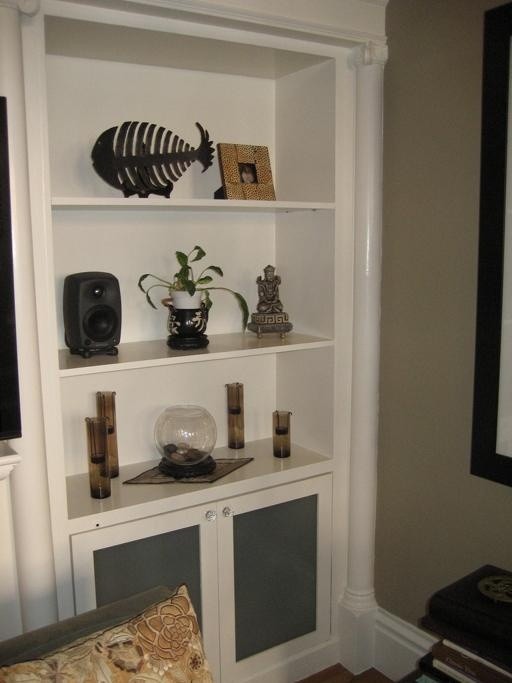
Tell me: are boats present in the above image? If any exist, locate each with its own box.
[63,270,123,359]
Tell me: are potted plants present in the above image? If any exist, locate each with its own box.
[217,142,278,201]
[472,3,511,490]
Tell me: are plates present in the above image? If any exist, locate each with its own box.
[18,0,388,682]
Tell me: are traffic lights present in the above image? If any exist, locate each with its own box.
[2,583,221,682]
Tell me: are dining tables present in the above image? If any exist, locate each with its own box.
[141,247,249,351]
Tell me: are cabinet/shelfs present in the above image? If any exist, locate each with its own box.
[95,389,119,478]
[85,416,111,499]
[271,409,292,457]
[226,382,245,448]
